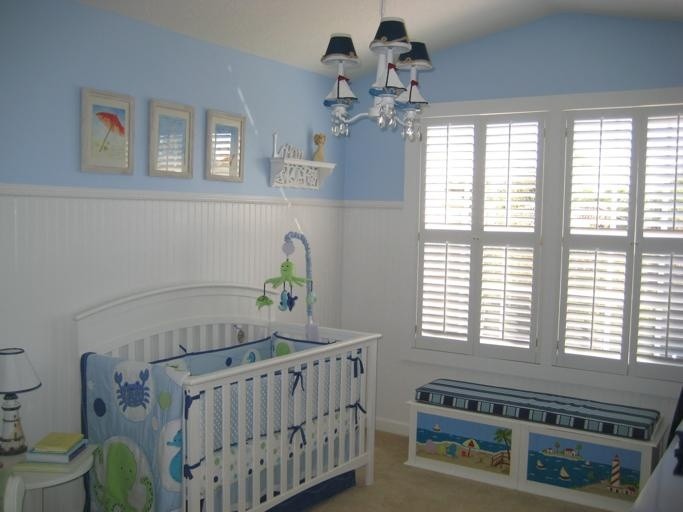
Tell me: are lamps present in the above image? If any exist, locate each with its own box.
[0,346,43,456]
[317,0,434,145]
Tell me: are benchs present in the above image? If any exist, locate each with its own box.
[402,376,672,512]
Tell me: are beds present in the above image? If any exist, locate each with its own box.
[79,313,380,512]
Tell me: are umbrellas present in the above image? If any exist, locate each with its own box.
[463,439,479,457]
[95,109,126,153]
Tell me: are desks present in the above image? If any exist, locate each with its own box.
[0,449,93,511]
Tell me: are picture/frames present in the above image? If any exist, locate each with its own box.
[77,85,247,184]
